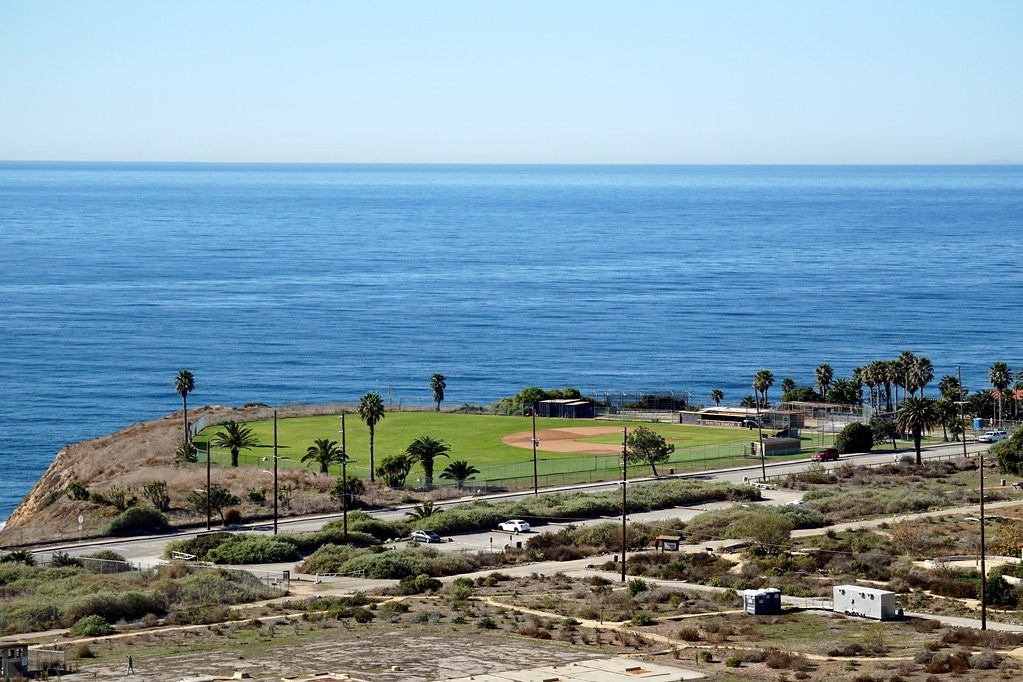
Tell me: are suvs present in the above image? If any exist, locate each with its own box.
[811,447,839,462]
[979,430,1008,443]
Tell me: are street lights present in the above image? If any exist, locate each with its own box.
[744,421,768,481]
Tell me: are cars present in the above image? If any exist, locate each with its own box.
[411,529,441,544]
[498,520,532,532]
